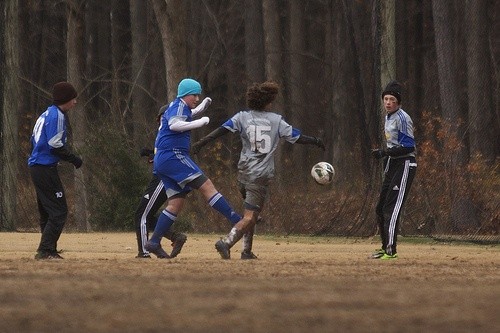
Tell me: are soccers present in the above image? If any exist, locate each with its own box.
[310,161,335,186]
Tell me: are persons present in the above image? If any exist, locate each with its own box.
[369,80,419,264]
[131,102,187,259]
[142,78,261,257]
[29,81,82,261]
[191,80,324,261]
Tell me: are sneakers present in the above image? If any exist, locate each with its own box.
[370,247,397,260]
[170,233,187,257]
[37,251,64,259]
[241,251,257,259]
[145,240,170,258]
[216,240,230,260]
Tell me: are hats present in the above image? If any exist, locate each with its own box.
[382,82,402,104]
[177,79,201,98]
[52,81,77,106]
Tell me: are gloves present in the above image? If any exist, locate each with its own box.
[371,148,388,160]
[73,156,83,169]
[315,136,327,151]
